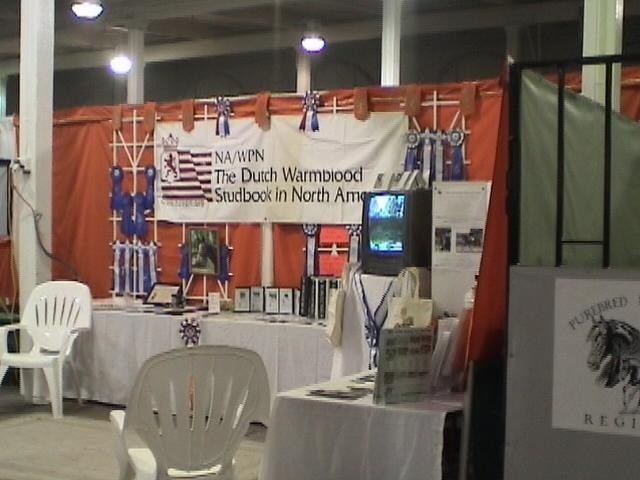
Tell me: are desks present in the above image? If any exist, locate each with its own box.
[22,305,331,423]
[257,371,462,480]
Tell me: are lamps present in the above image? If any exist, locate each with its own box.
[110,51,132,74]
[71,0,102,20]
[299,18,326,52]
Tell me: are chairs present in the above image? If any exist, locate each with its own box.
[1,281,96,421]
[108,345,270,480]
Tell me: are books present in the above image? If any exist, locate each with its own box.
[300,276,343,319]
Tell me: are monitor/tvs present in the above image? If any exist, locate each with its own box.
[361,190,432,277]
